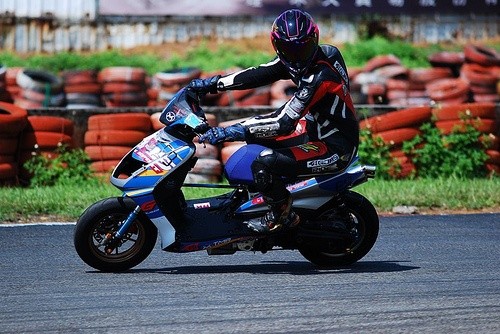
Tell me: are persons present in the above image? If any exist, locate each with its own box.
[188,9,360,233]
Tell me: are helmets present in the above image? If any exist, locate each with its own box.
[270,11,319,77]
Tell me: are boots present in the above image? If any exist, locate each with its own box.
[248,196,299,233]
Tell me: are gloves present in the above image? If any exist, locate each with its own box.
[188,74,222,98]
[198,121,245,146]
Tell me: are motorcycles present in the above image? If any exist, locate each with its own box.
[72,81,380,272]
